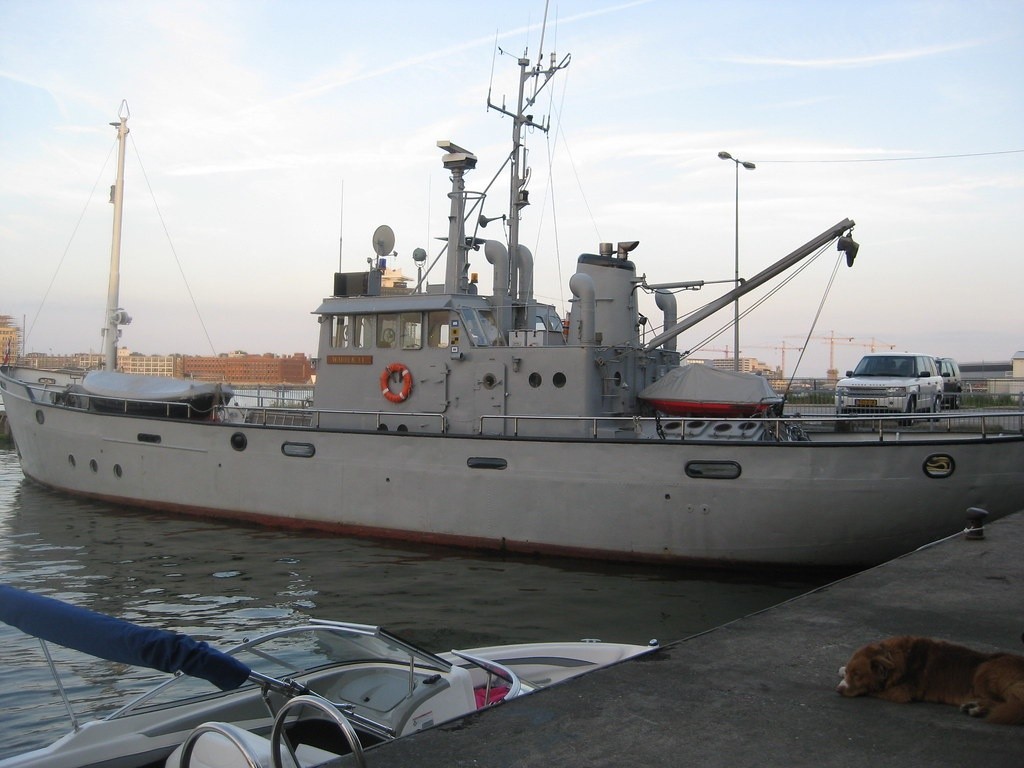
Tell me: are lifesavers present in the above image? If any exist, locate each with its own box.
[380,362,413,403]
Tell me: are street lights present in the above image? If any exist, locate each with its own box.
[716,151,756,374]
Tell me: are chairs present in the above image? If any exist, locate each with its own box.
[164,722,341,768]
[898,361,909,371]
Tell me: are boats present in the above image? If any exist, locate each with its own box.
[1,580,665,768]
[81,369,235,421]
[0,1,1024,571]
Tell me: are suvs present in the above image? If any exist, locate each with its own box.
[834,353,945,426]
[934,357,963,409]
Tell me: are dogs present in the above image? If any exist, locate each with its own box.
[834,635,1024,725]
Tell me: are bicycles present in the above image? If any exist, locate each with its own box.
[760,402,811,442]
